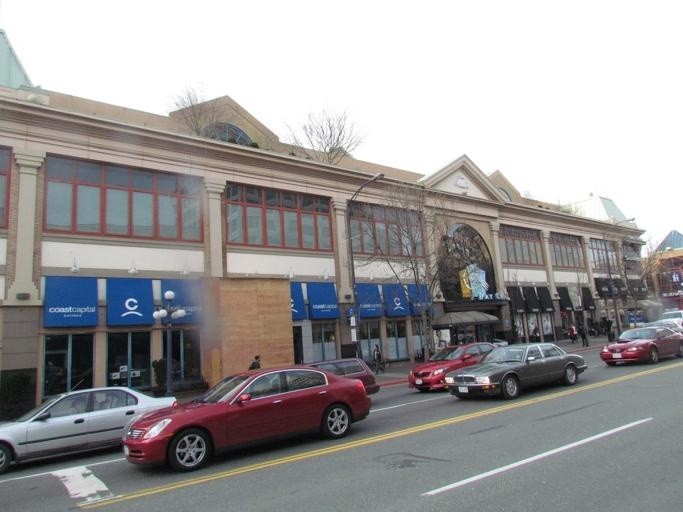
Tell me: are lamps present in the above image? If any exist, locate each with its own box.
[69,254,139,277]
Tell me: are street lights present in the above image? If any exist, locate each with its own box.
[151,287,186,398]
[344,170,387,359]
[601,216,637,337]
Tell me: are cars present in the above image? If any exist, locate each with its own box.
[121,363,372,472]
[598,310,682,366]
[0,386,178,475]
[45,350,205,396]
[406,341,496,393]
[441,343,587,402]
[297,358,380,395]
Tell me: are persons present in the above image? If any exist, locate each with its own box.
[248,355,260,370]
[250,377,272,399]
[74,396,87,413]
[567,323,579,343]
[371,344,383,375]
[577,323,589,347]
[433,330,449,352]
